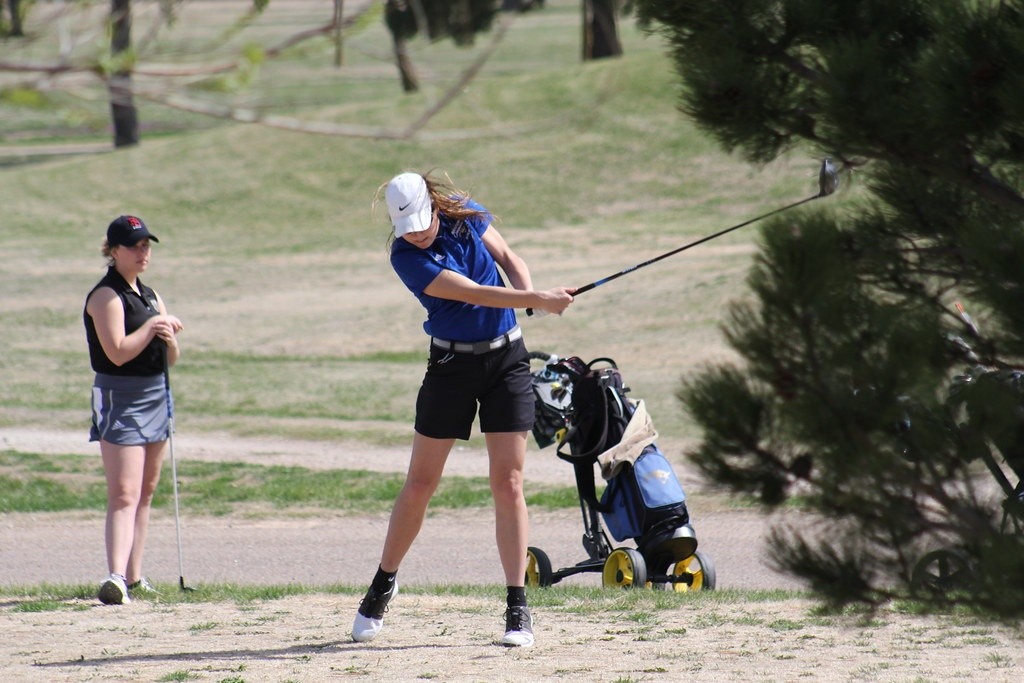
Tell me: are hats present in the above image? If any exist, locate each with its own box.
[107,215,159,248]
[384,172,433,238]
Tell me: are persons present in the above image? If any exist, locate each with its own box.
[84,215,186,604]
[352,172,580,648]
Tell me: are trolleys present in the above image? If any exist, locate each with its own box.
[524,350,716,592]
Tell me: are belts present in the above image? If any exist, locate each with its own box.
[428,324,523,356]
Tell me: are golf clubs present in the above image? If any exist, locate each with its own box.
[160,331,197,593]
[525,160,837,319]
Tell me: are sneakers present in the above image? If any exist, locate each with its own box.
[500,606,534,648]
[124,580,163,601]
[97,578,131,605]
[350,578,399,642]
